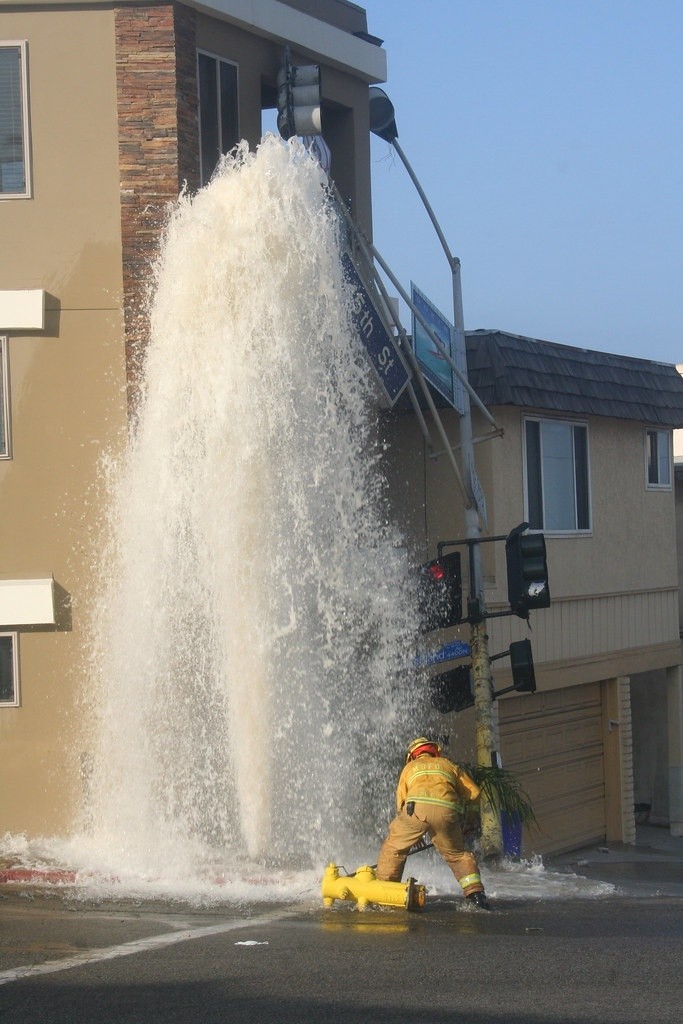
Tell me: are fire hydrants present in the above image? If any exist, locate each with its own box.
[319,863,425,910]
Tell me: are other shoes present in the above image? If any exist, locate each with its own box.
[473,892,496,909]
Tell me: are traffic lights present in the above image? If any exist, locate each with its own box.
[507,528,550,615]
[510,640,537,692]
[431,667,470,712]
[420,552,465,629]
[275,59,322,140]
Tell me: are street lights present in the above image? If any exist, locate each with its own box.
[369,84,502,862]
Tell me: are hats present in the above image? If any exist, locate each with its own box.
[406,737,442,763]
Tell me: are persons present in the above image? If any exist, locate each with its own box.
[375,736,496,911]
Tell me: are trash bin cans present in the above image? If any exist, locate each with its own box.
[459,805,522,863]
